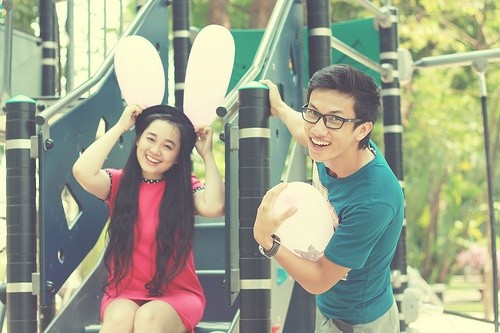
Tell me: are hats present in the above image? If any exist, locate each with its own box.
[135,104,198,152]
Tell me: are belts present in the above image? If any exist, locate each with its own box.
[332,319,353,333]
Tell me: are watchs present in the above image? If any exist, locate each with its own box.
[258,234,281,260]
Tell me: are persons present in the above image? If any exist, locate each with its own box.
[252,62,407,333]
[71,101,226,333]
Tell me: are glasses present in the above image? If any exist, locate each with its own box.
[301,104,365,129]
